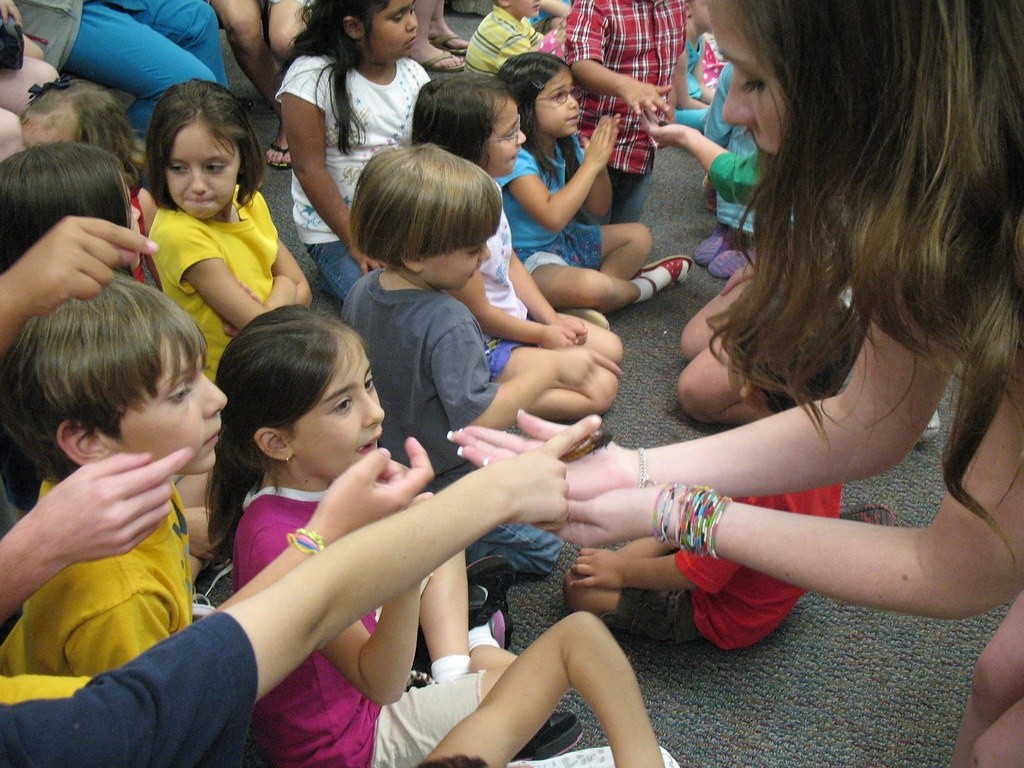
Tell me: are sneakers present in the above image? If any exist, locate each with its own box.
[511,712,584,761]
[466,554,515,651]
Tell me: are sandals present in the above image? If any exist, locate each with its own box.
[631,256,693,295]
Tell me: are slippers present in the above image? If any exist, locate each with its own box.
[429,33,468,55]
[422,49,466,72]
[268,143,292,170]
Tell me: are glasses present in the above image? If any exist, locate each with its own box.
[534,85,582,104]
[482,114,520,141]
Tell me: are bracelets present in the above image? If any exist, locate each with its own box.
[652,481,733,561]
[287,528,326,555]
[638,446,649,488]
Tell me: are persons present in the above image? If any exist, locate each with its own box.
[1,137,231,628]
[422,610,667,767]
[441,0,1024,766]
[206,307,579,768]
[3,412,600,768]
[637,0,942,439]
[1,0,685,418]
[0,272,434,680]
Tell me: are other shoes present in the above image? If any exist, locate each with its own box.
[694,222,737,266]
[835,503,895,527]
[708,245,756,278]
